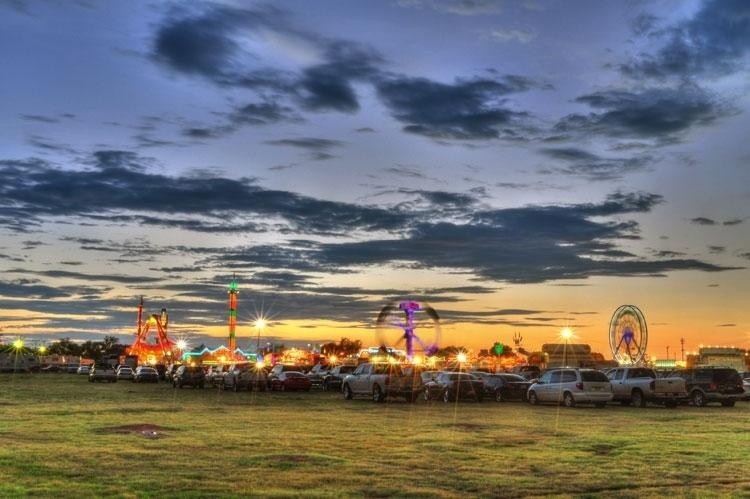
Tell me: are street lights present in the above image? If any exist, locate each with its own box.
[560,325,575,367]
[254,318,268,352]
